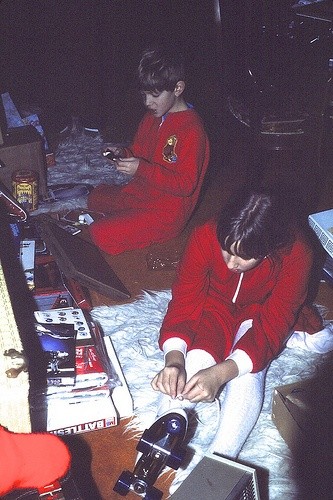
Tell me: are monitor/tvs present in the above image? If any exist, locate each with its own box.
[39,221,130,301]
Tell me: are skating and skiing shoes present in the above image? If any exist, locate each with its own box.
[112,409,188,500]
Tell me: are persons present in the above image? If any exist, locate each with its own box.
[112,189,325,500]
[88,46,210,256]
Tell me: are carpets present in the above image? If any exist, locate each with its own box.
[27,132,133,217]
[90,288,333,500]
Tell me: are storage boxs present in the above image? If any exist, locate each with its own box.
[0,125,48,195]
[272,376,333,459]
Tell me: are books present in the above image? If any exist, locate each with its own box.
[30,322,123,437]
[308,209,333,283]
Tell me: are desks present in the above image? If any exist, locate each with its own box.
[308,208,333,305]
